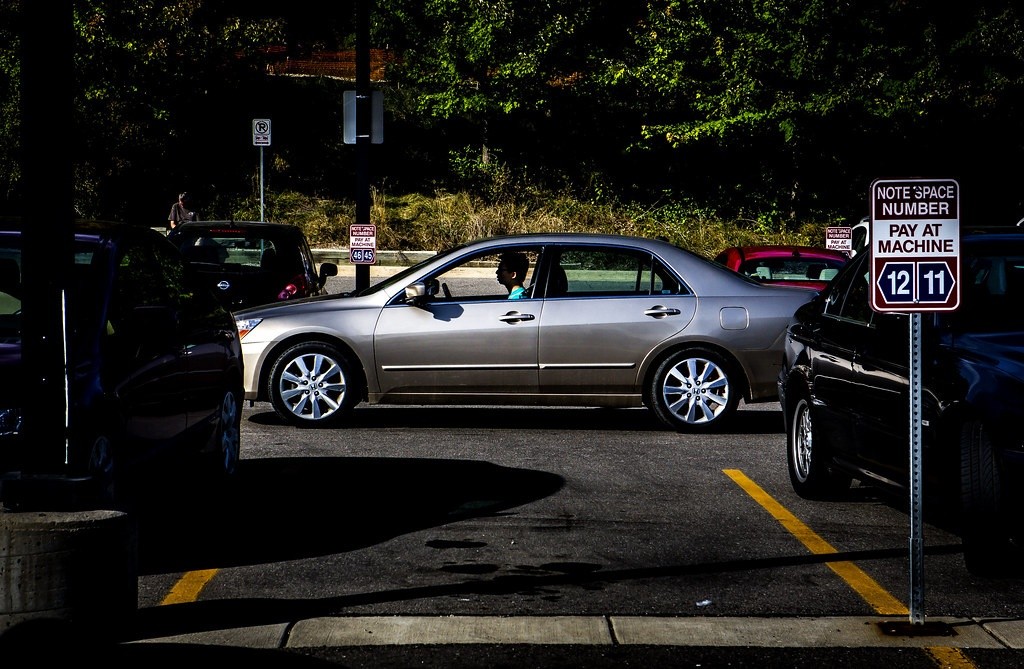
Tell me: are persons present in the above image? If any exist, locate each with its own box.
[167,192,199,228]
[495,253,533,301]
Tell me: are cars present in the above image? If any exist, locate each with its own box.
[709,245,869,293]
[163,220,338,323]
[0,219,246,507]
[847,218,871,263]
[231,226,822,431]
[774,241,1024,569]
[957,212,1023,263]
